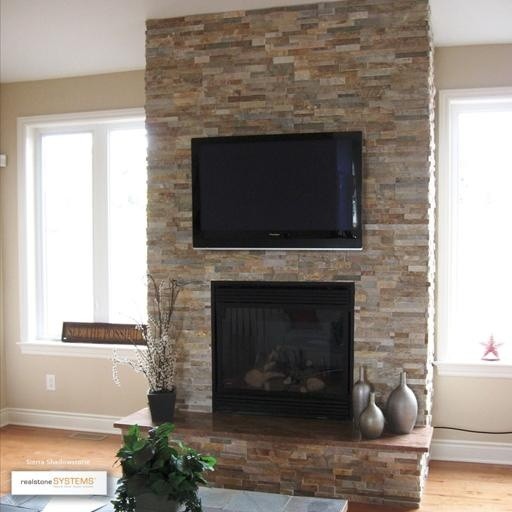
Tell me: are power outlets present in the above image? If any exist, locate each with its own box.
[46,374,55,391]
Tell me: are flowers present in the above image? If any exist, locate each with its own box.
[109,273,207,395]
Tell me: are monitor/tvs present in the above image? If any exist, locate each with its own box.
[192,131,362,248]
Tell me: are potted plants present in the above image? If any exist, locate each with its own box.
[110,422,217,512]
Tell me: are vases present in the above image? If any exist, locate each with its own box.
[351,365,419,440]
[147,388,177,425]
